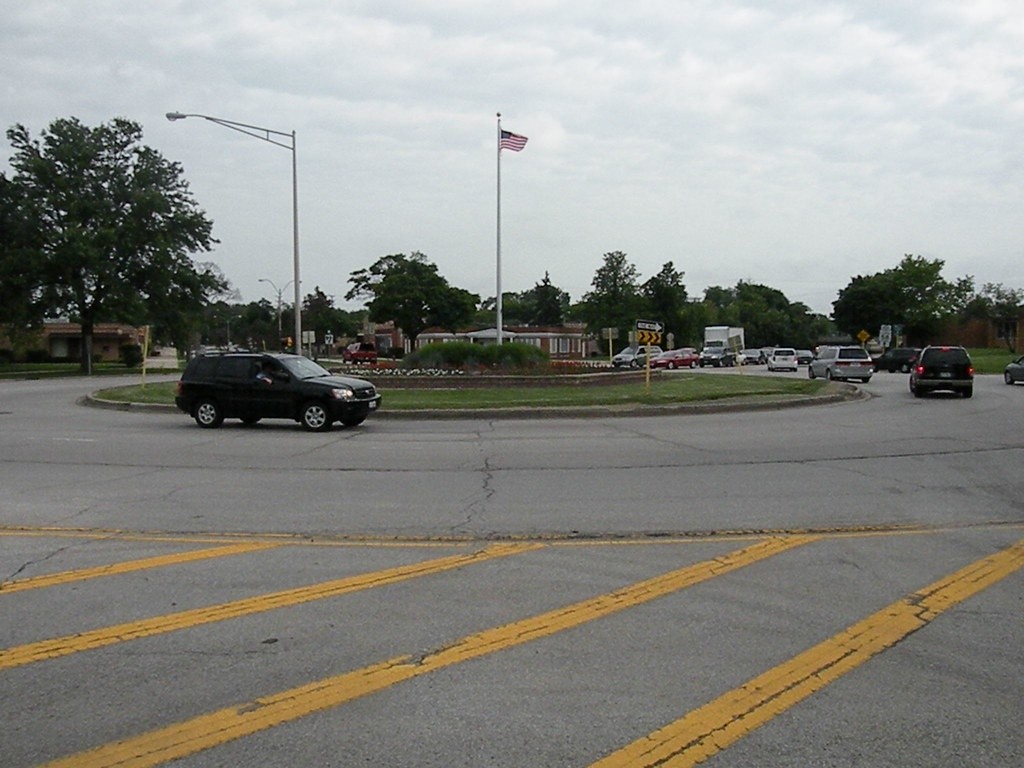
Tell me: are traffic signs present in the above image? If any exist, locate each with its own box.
[638,331,661,343]
[635,319,664,333]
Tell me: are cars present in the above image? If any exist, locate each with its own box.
[649,348,698,370]
[796,350,813,365]
[611,346,663,367]
[203,345,220,356]
[872,348,922,374]
[700,347,734,368]
[1004,355,1024,385]
[677,348,697,356]
[767,348,798,372]
[741,349,768,366]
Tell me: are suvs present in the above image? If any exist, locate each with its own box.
[909,345,974,398]
[343,341,377,365]
[808,345,874,383]
[175,351,382,432]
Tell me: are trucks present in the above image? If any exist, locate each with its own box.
[703,326,745,365]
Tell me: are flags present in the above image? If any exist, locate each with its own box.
[499,128,528,153]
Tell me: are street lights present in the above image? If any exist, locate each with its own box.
[166,111,302,356]
[259,279,302,354]
[226,315,242,342]
